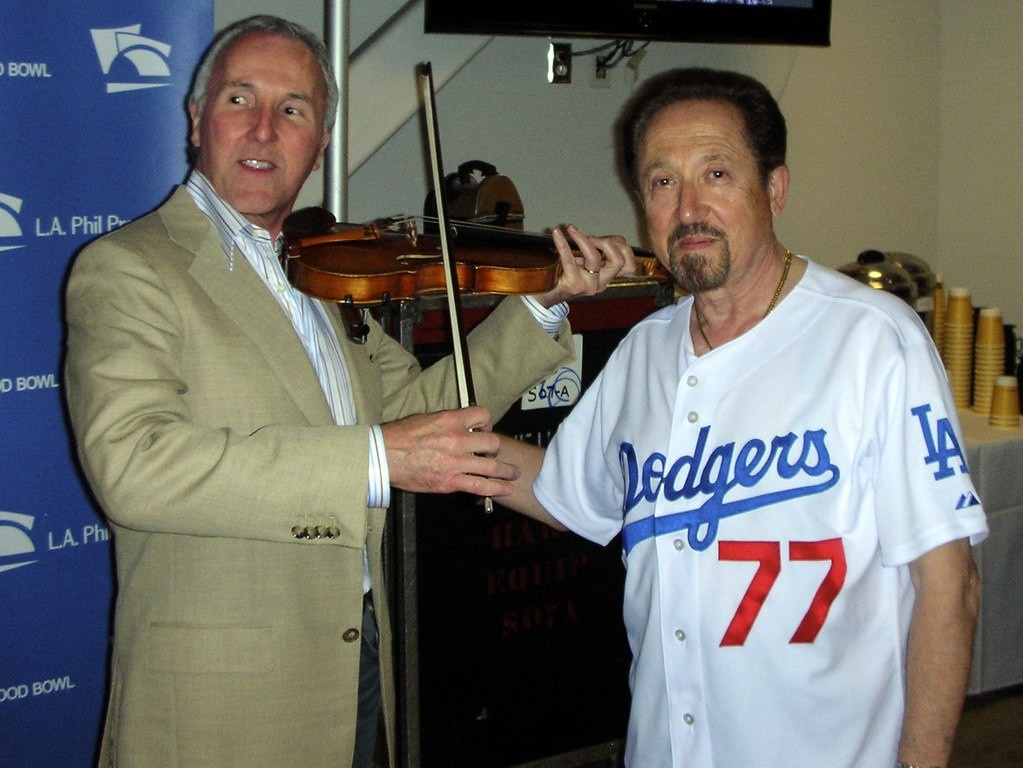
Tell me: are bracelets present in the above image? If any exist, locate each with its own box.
[896,761,919,768]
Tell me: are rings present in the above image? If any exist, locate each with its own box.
[584,267,599,274]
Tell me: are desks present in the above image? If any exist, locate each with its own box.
[953,405,1023,697]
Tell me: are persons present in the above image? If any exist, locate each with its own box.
[65,15,637,767]
[487,65,989,768]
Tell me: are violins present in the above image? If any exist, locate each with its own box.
[282,212,673,305]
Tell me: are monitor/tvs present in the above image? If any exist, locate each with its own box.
[419,0,833,50]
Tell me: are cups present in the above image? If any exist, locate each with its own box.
[933,271,1019,432]
